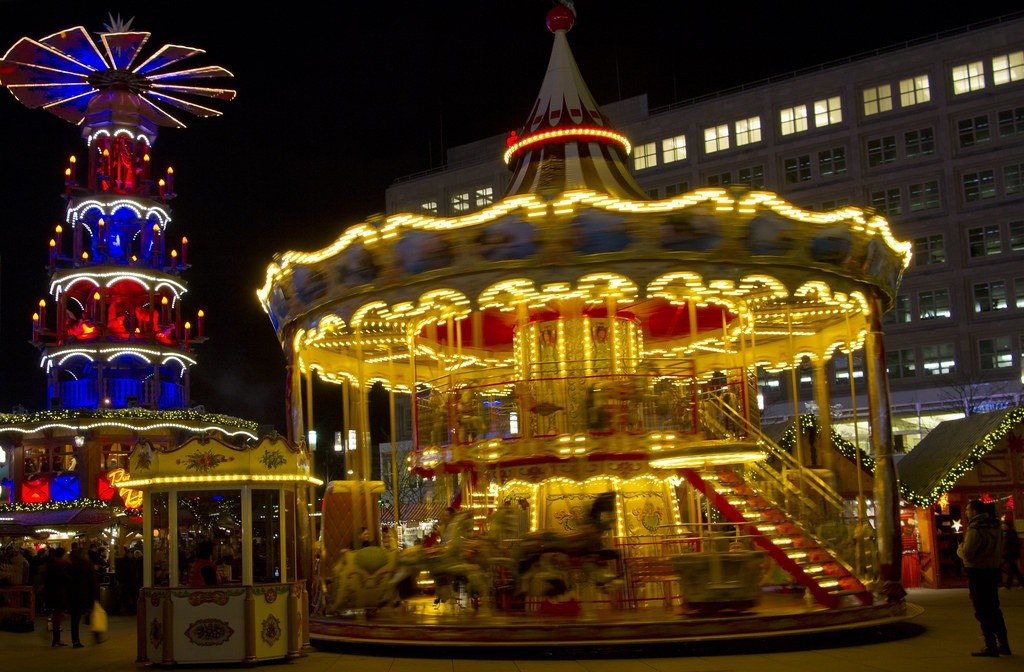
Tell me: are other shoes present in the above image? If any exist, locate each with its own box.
[999,586,1010,592]
[98,636,110,644]
[997,644,1012,656]
[73,641,84,648]
[971,646,999,657]
[1016,585,1024,591]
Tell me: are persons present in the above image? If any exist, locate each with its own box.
[25,456,62,472]
[214,529,235,581]
[955,498,1013,658]
[190,541,222,586]
[999,521,1024,589]
[439,506,462,599]
[0,541,143,647]
[349,527,371,548]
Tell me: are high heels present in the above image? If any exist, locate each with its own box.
[52,640,68,647]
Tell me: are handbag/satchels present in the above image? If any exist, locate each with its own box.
[91,600,108,633]
